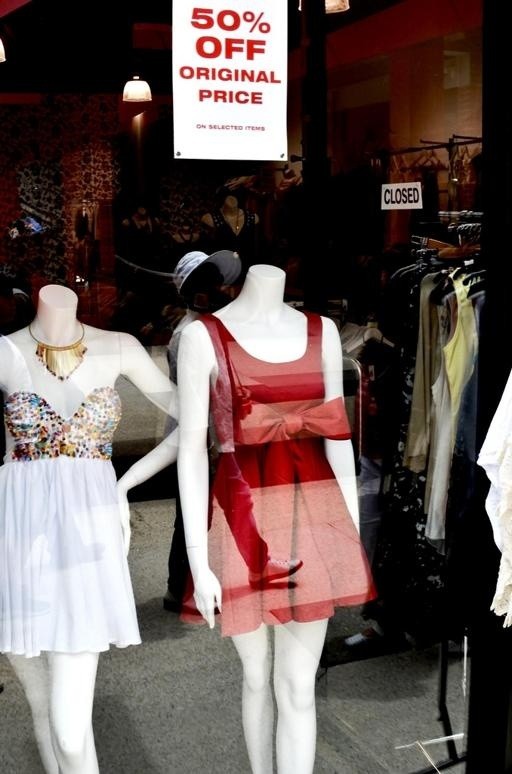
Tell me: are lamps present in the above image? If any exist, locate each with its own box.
[122,75,153,103]
[298,0,351,15]
[0,38,7,63]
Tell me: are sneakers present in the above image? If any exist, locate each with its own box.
[250,560,303,590]
[164,591,221,617]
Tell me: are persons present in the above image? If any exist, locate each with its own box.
[162,246,305,616]
[174,262,382,774]
[201,194,263,284]
[1,281,180,774]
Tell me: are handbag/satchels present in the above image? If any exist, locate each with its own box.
[237,389,252,420]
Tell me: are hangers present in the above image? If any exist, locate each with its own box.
[336,132,488,353]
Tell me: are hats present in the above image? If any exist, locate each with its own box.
[172,250,242,301]
